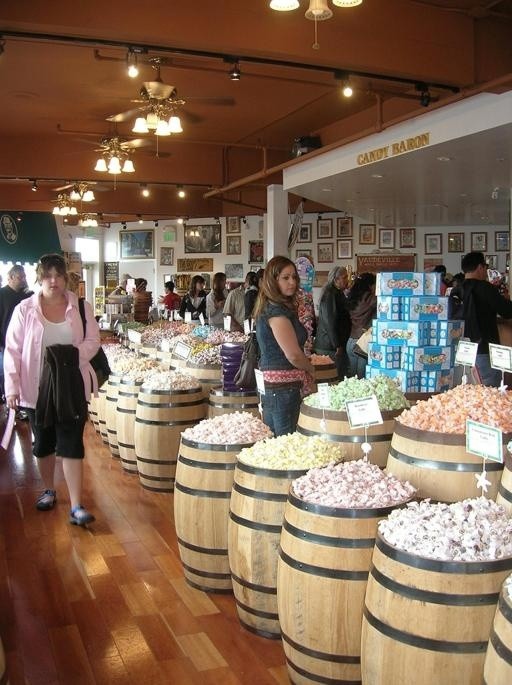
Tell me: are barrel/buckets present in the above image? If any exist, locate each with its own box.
[173,431,276,594]
[134,381,206,493]
[383,415,507,505]
[92,392,101,437]
[495,438,512,516]
[208,382,263,424]
[139,342,155,359]
[169,354,187,372]
[360,522,512,685]
[226,451,345,642]
[86,398,93,424]
[308,361,339,389]
[104,372,127,458]
[292,391,408,469]
[275,482,418,685]
[153,344,172,363]
[127,338,137,353]
[115,376,144,473]
[183,361,223,386]
[98,377,108,441]
[480,571,512,684]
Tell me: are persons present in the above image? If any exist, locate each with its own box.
[313,266,351,381]
[431,265,448,296]
[109,273,135,297]
[244,269,265,319]
[450,252,512,387]
[3,254,96,526]
[222,272,257,333]
[445,273,465,304]
[159,281,181,319]
[346,271,377,380]
[254,256,316,437]
[206,273,229,329]
[186,226,220,253]
[179,275,208,320]
[0,265,35,421]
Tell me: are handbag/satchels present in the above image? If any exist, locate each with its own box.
[79,295,112,392]
[352,328,371,361]
[234,319,260,390]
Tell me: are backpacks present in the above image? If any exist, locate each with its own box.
[448,280,482,345]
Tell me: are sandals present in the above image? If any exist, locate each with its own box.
[36,490,56,510]
[70,504,95,525]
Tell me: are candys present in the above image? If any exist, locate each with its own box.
[292,456,418,509]
[396,381,512,434]
[504,573,511,601]
[236,431,346,471]
[378,495,512,563]
[182,410,273,446]
[308,353,334,365]
[99,318,251,391]
[303,372,411,412]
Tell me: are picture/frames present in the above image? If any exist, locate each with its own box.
[423,258,442,275]
[177,258,212,272]
[336,217,354,237]
[297,222,312,243]
[447,232,466,254]
[399,228,416,250]
[293,249,311,256]
[248,240,266,264]
[424,233,442,255]
[505,253,511,274]
[316,218,333,240]
[314,271,332,287]
[359,222,378,246]
[50,174,111,194]
[224,263,244,279]
[470,231,487,252]
[159,246,175,268]
[316,242,334,263]
[339,239,354,259]
[183,225,222,254]
[378,227,396,250]
[496,231,510,251]
[118,229,153,259]
[225,235,243,255]
[226,217,241,232]
[249,266,262,273]
[485,255,498,271]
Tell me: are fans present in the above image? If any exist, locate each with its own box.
[60,134,171,159]
[77,79,236,124]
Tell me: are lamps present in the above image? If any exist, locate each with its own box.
[67,188,96,216]
[131,108,185,160]
[49,195,79,228]
[268,1,364,50]
[94,155,137,191]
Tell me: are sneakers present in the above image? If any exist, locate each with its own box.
[16,410,28,420]
